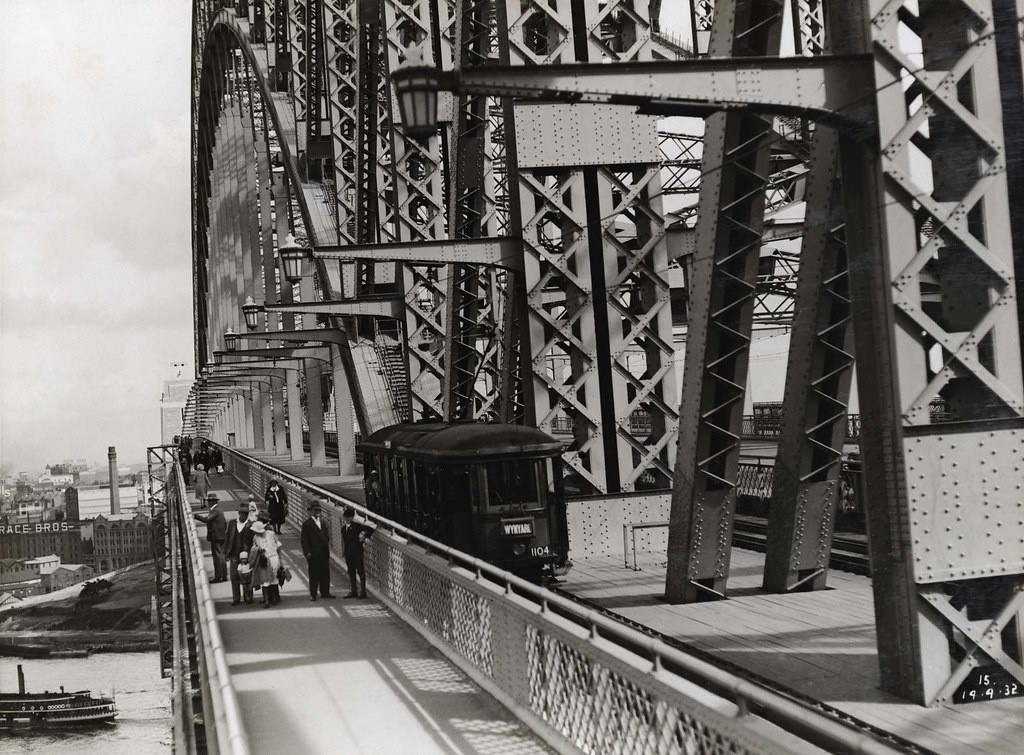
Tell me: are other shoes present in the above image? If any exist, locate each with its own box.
[201,506,204,509]
[244,599,255,604]
[278,531,282,535]
[231,599,241,605]
[309,595,316,601]
[259,599,264,603]
[209,576,227,583]
[357,593,367,600]
[203,505,207,508]
[343,592,357,599]
[321,594,336,598]
[264,602,270,608]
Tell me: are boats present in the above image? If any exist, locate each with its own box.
[0,664,120,728]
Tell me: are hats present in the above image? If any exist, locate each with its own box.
[306,500,323,511]
[240,551,249,559]
[235,502,249,511]
[250,521,265,533]
[204,492,220,500]
[268,477,279,484]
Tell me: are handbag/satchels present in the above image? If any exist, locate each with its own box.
[282,568,292,581]
[217,465,224,473]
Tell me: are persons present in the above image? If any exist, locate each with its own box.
[301,500,337,602]
[177,434,223,509]
[192,493,228,584]
[223,477,291,610]
[338,508,376,601]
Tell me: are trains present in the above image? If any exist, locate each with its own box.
[352,416,577,592]
